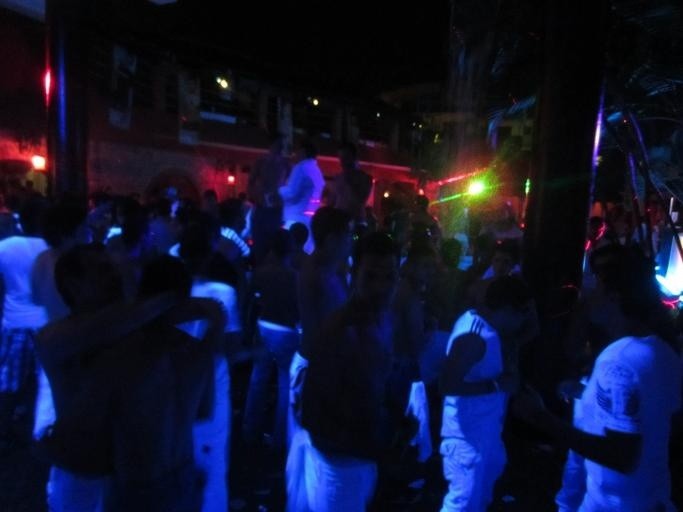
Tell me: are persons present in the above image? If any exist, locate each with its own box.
[275,136,326,259]
[0,167,681,511]
[315,140,373,239]
[246,130,291,265]
